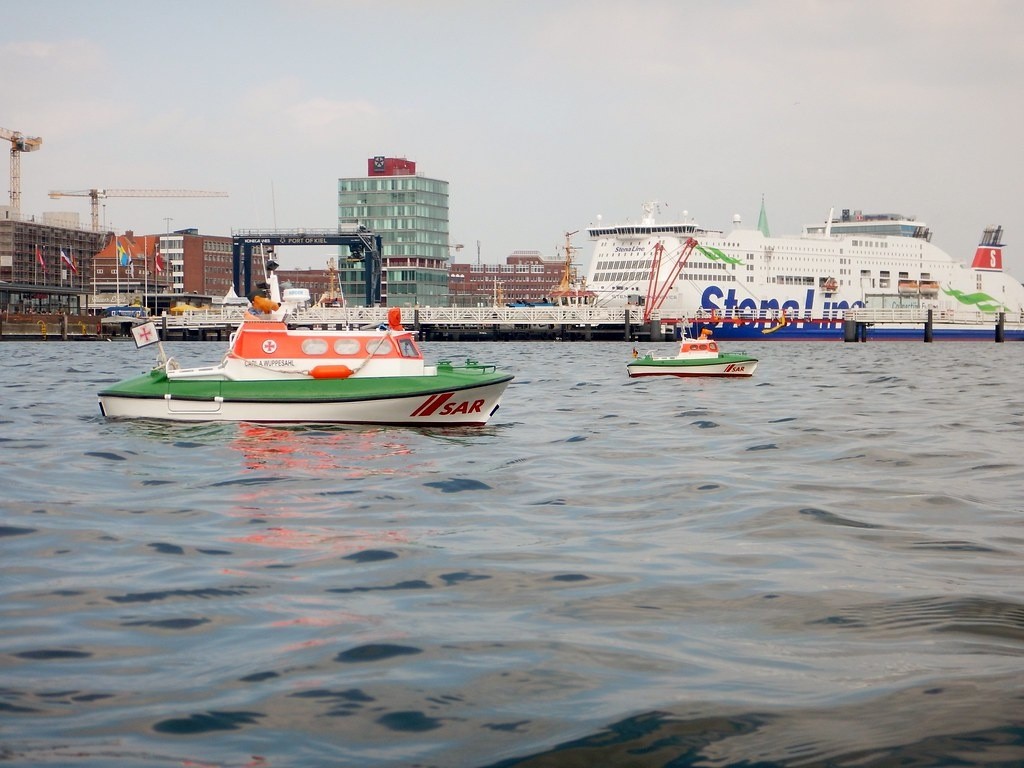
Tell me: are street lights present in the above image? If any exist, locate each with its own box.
[163,217,173,287]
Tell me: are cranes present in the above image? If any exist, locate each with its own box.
[0,127,42,208]
[48,187,229,234]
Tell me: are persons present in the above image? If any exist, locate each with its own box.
[132,311,141,318]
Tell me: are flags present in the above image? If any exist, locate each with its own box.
[757,200,770,238]
[60,248,80,274]
[35,248,47,272]
[116,238,164,279]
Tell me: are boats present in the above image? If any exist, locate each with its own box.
[625,310,760,377]
[96,288,516,426]
